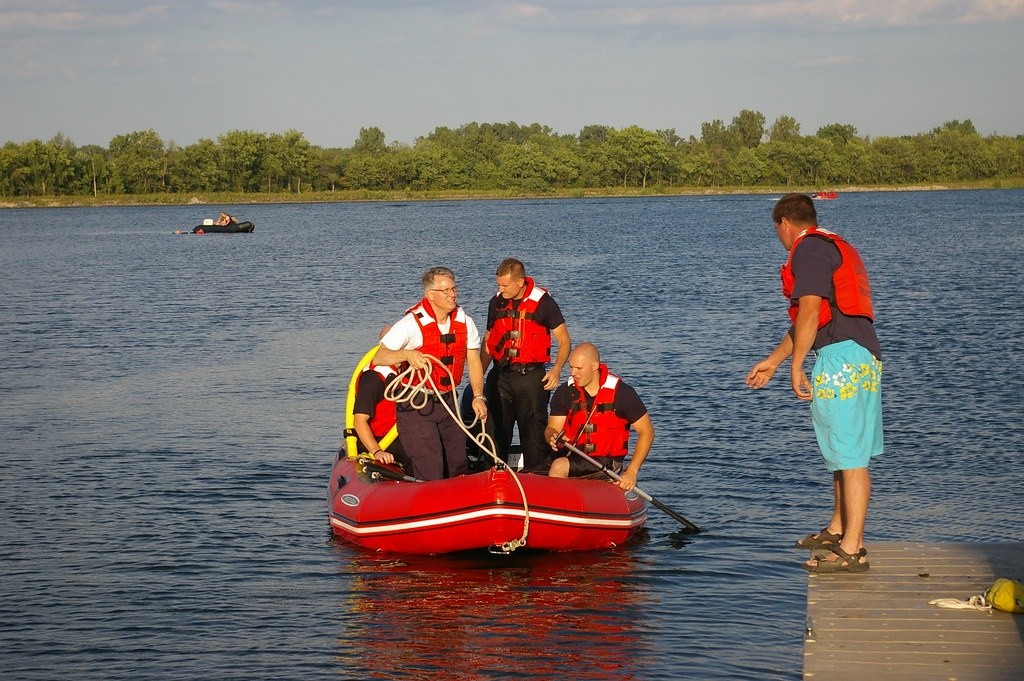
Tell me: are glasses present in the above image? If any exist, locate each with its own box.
[429,286,460,294]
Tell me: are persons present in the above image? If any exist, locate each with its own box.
[353,324,414,478]
[216,212,234,225]
[745,193,883,571]
[544,343,654,491]
[372,267,488,482]
[479,258,571,477]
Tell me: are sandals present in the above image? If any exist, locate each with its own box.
[795,529,843,549]
[804,546,869,572]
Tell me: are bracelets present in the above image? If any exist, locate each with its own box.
[472,393,486,401]
[373,449,383,456]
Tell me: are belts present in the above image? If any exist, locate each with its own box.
[492,360,545,375]
[398,386,458,403]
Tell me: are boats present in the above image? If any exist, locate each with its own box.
[327,440,648,556]
[192,221,257,233]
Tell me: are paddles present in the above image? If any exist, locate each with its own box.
[364,462,425,482]
[554,429,708,533]
